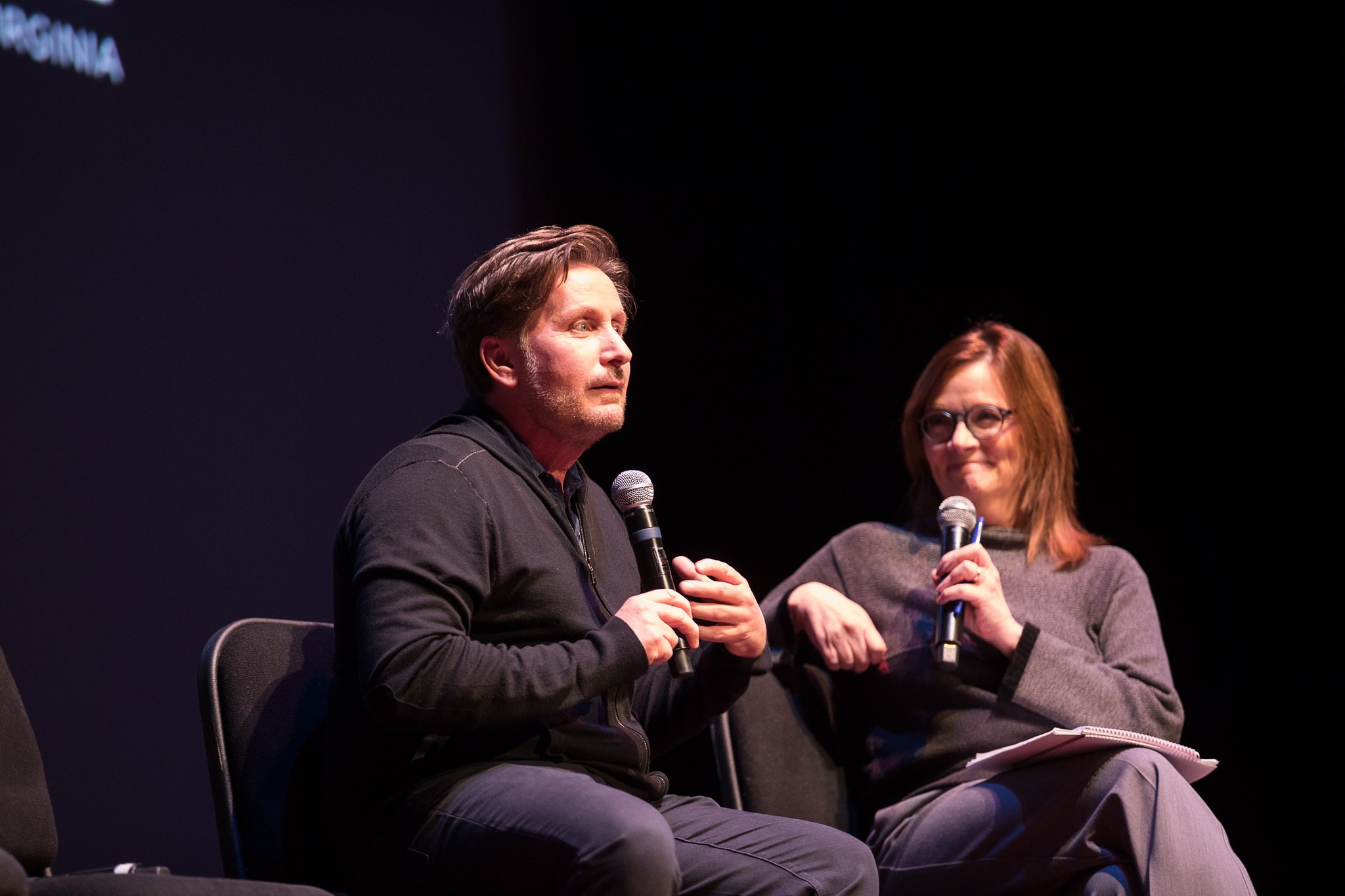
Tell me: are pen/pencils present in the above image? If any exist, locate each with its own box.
[952,516,985,615]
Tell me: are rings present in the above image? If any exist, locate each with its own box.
[973,566,986,581]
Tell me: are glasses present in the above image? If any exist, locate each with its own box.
[912,404,1015,447]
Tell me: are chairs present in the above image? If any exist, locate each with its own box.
[712,654,871,839]
[198,618,342,891]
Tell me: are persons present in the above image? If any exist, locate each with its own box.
[329,223,881,896]
[760,321,1256,896]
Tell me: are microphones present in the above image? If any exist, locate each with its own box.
[938,495,977,669]
[611,470,697,679]
